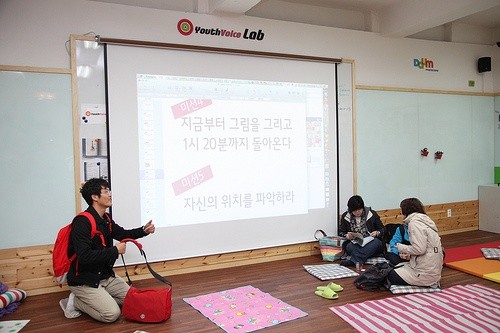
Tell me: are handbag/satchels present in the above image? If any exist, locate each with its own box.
[121,284,172,323]
[384,222,402,242]
[354,262,393,290]
[314,229,348,261]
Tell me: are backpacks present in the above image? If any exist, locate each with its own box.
[52,212,111,286]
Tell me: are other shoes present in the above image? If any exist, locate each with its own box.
[12,288,26,299]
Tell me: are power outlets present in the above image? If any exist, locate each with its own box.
[447,209,452,218]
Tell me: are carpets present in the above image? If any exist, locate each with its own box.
[182,285,309,333]
[329,284,500,333]
[303,264,360,280]
[0,320,30,333]
[439,241,500,284]
[367,256,388,264]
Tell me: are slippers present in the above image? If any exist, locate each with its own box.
[317,282,343,292]
[59,298,82,318]
[314,288,339,299]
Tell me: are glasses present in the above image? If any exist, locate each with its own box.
[100,190,112,195]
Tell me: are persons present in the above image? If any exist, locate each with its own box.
[388,198,443,288]
[385,218,412,264]
[338,195,386,267]
[58,178,155,322]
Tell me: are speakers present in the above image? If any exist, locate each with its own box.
[477,56,492,73]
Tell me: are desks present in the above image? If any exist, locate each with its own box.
[478,184,500,234]
